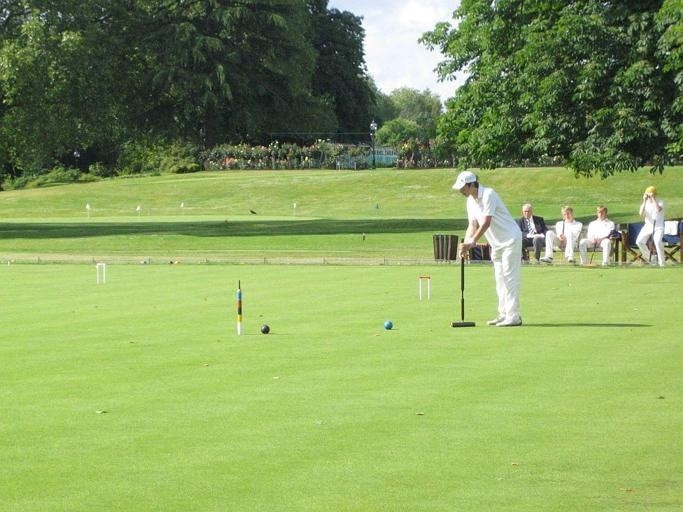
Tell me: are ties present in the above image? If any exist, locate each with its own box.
[527,219,534,234]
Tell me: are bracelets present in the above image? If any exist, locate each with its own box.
[468,239,476,247]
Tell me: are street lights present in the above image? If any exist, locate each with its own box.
[369,118,377,170]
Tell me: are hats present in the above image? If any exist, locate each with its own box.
[452,171,476,190]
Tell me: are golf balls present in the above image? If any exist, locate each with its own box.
[168,261,179,263]
[141,260,146,264]
[383,320,393,329]
[261,325,270,335]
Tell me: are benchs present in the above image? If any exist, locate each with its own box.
[523,218,682,264]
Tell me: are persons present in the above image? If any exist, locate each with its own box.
[576,206,616,268]
[512,204,548,265]
[635,186,667,268]
[450,170,523,327]
[539,206,583,263]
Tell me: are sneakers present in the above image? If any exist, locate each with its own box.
[659,261,665,267]
[601,260,608,265]
[487,312,506,325]
[540,256,552,263]
[568,256,575,262]
[495,316,522,326]
